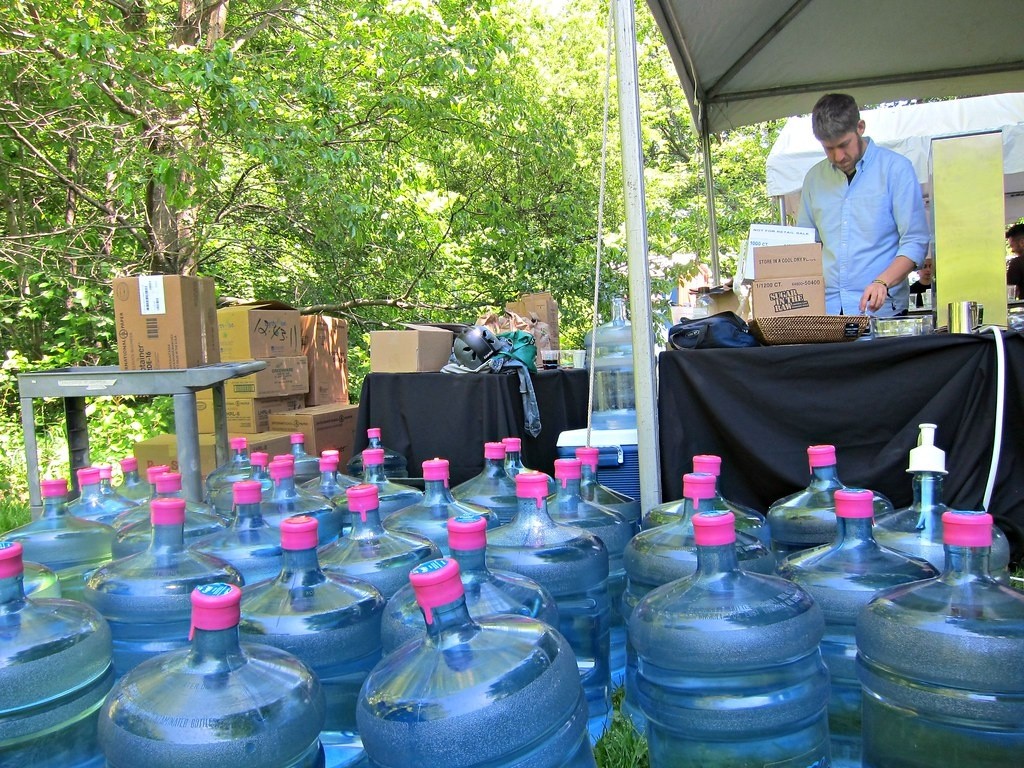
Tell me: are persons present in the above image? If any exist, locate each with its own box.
[909,258,933,308]
[1005,223,1024,300]
[796,93,930,319]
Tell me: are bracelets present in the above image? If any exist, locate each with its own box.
[872,280,889,290]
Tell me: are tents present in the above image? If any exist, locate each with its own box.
[614,0,1024,518]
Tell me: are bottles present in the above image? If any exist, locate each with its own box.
[584,298,657,430]
[1,426,1023,768]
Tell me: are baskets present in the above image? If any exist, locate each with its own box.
[756,314,869,342]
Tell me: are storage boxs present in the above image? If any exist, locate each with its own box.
[927,130,1007,329]
[557,428,641,526]
[113,273,359,493]
[506,292,559,367]
[370,322,454,373]
[740,224,825,318]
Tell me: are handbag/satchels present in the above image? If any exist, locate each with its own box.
[669,310,756,350]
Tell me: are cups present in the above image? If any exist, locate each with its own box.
[1007,285,1016,303]
[908,288,932,311]
[541,350,586,371]
[948,301,978,334]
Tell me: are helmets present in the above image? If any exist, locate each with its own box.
[455,325,503,367]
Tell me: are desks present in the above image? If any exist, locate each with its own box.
[658,329,1024,563]
[355,369,589,491]
[909,300,1024,316]
